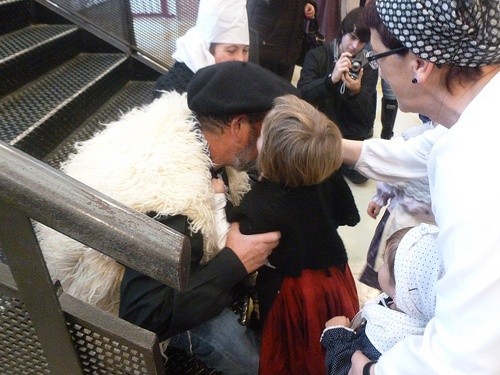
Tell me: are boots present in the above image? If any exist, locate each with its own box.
[381,99,398,140]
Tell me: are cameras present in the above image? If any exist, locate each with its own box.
[348,60,362,80]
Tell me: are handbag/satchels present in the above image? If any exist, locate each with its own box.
[296,18,325,67]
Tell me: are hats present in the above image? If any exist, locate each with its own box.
[375,0,500,67]
[186,61,299,113]
[394,223,441,320]
[192,0,249,45]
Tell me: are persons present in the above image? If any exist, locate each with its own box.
[152,0,250,101]
[246,0,367,84]
[211,94,361,375]
[297,6,379,184]
[357,114,439,294]
[320,222,446,375]
[342,0,500,375]
[381,78,398,139]
[33,60,301,375]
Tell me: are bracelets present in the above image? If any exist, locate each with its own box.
[362,361,377,375]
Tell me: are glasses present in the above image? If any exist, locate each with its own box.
[365,47,409,70]
